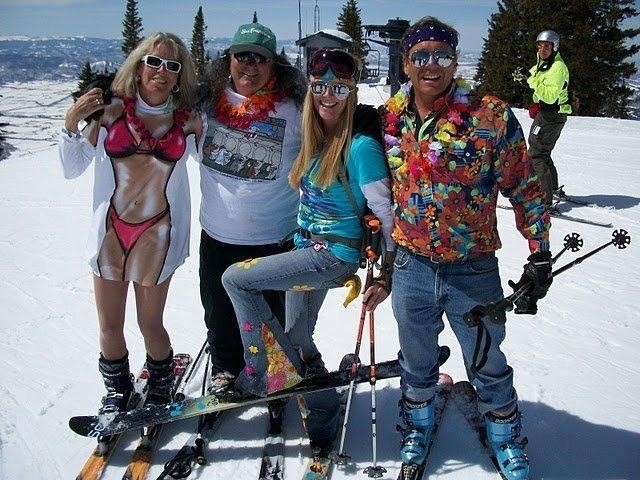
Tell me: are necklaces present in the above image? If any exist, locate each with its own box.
[142,90,167,102]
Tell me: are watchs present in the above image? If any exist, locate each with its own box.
[60,126,80,140]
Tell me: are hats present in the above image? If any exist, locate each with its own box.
[229,23,276,58]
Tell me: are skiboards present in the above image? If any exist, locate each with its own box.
[495,185,614,229]
[67,333,537,480]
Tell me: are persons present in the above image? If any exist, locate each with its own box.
[196,22,303,434]
[512,29,573,208]
[58,32,203,453]
[377,15,554,480]
[221,49,398,459]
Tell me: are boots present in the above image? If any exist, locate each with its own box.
[483,404,531,480]
[141,347,175,440]
[98,352,135,443]
[396,393,436,465]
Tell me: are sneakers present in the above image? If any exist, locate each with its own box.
[226,363,306,402]
[204,371,236,419]
[310,422,339,447]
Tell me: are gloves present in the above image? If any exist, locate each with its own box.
[508,251,553,315]
[519,67,532,78]
[83,78,111,123]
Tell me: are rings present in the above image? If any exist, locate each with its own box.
[95,98,100,105]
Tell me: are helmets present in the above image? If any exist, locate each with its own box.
[535,30,559,52]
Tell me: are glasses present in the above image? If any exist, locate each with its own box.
[234,51,266,63]
[141,55,182,74]
[309,50,357,101]
[408,49,454,67]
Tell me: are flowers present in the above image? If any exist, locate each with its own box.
[237,257,256,270]
[289,285,315,292]
[241,323,305,395]
[384,72,470,181]
[211,69,291,129]
